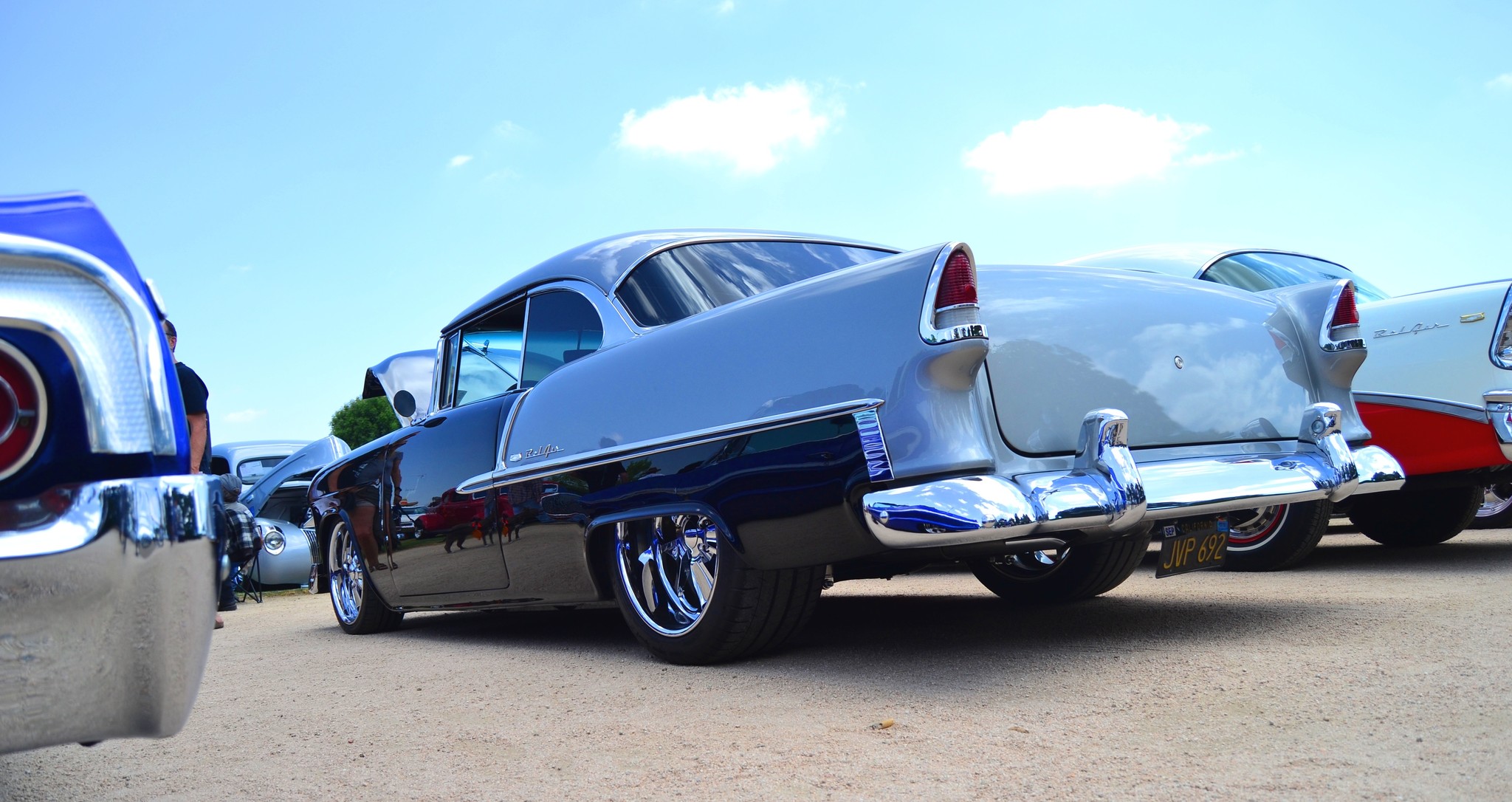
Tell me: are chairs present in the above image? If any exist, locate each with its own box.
[229,508,264,605]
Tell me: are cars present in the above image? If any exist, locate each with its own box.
[208,436,353,591]
[0,197,221,745]
[394,505,429,539]
[1162,253,1511,561]
[307,226,1408,663]
[413,486,517,536]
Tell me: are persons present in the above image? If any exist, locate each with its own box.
[325,440,408,573]
[215,472,263,611]
[579,438,629,520]
[483,488,497,533]
[162,319,224,629]
[507,478,556,528]
[638,467,665,480]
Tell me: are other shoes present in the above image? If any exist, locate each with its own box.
[217,602,238,611]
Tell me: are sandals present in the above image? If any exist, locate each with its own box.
[369,566,375,572]
[393,562,398,569]
[375,563,388,571]
[215,617,224,629]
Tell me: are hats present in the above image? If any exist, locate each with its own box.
[220,473,242,495]
